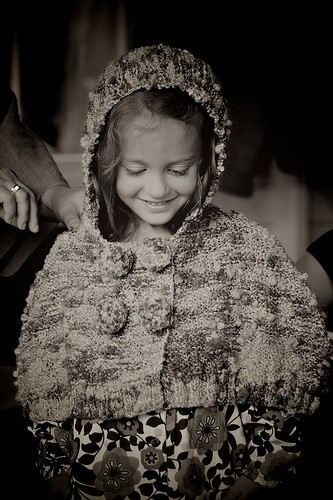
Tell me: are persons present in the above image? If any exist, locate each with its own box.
[0,104,84,232]
[14,45,328,500]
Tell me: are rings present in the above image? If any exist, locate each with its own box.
[11,185,19,193]
